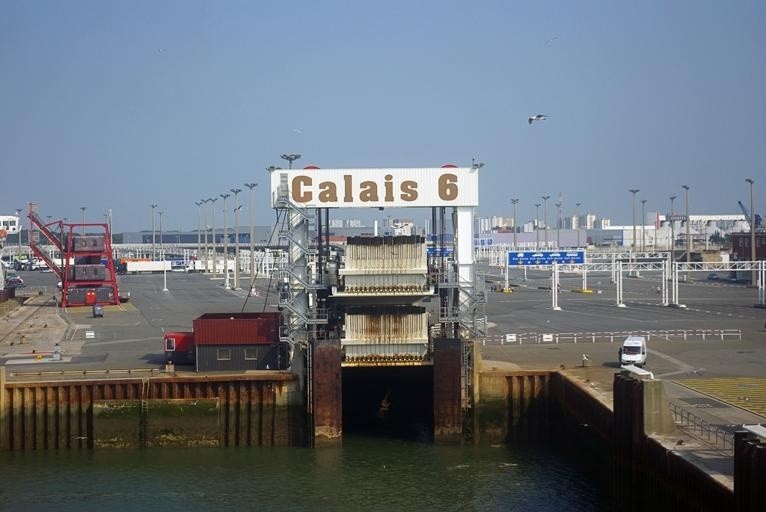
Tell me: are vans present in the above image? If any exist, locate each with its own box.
[621,336,647,367]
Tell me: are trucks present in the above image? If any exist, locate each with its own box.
[118,261,172,274]
[4,259,74,269]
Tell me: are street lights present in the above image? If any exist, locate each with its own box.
[45,215,51,258]
[157,211,164,247]
[230,184,242,290]
[194,196,218,281]
[510,184,691,280]
[149,204,157,260]
[81,207,87,236]
[16,208,21,258]
[280,153,302,168]
[243,181,259,297]
[220,192,230,290]
[744,177,758,288]
[62,217,68,234]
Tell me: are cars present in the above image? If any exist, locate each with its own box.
[4,269,24,289]
[708,273,719,280]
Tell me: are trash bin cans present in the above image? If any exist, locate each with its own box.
[93,304,104,317]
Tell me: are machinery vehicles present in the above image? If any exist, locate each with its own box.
[26,211,121,308]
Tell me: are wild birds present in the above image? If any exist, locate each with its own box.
[471,156,486,169]
[233,205,244,212]
[528,113,552,124]
